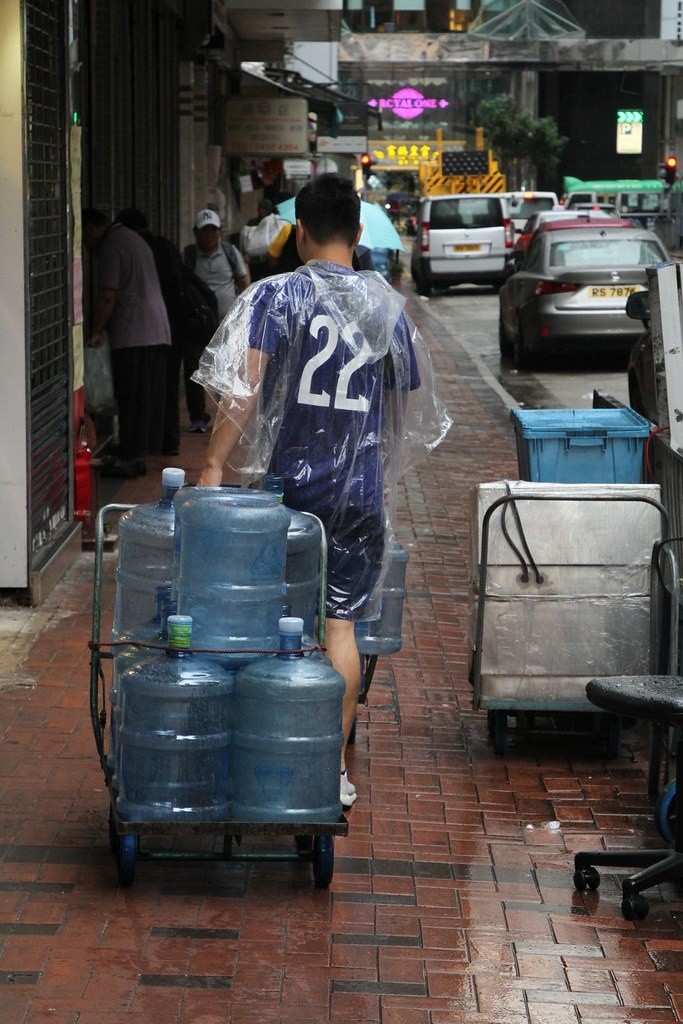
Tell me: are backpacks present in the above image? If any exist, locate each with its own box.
[247,213,286,258]
[155,251,218,347]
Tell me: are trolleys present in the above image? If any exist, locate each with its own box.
[91,501,350,891]
[467,494,672,760]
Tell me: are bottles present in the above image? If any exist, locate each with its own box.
[108,467,345,820]
[354,540,409,653]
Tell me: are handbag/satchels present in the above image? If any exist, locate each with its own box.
[84,335,113,414]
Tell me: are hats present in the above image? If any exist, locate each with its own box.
[194,209,221,230]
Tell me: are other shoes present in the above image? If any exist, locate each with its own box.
[164,447,179,455]
[189,418,207,433]
[112,462,146,480]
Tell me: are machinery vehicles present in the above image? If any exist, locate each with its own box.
[419,126,506,195]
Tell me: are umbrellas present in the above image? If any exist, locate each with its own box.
[275,196,405,253]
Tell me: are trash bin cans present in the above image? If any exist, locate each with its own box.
[510,407,649,484]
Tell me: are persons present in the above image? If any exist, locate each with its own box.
[81,208,172,477]
[189,173,454,810]
[180,208,250,434]
[117,208,184,456]
[243,198,375,278]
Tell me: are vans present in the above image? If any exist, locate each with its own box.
[406,194,514,296]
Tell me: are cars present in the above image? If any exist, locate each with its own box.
[512,211,636,271]
[571,203,621,219]
[498,227,672,372]
[625,288,659,427]
[500,190,559,241]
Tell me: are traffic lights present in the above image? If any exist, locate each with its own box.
[366,159,377,178]
[667,156,677,182]
[361,153,370,174]
[658,162,667,179]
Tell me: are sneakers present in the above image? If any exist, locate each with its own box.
[339,770,358,808]
